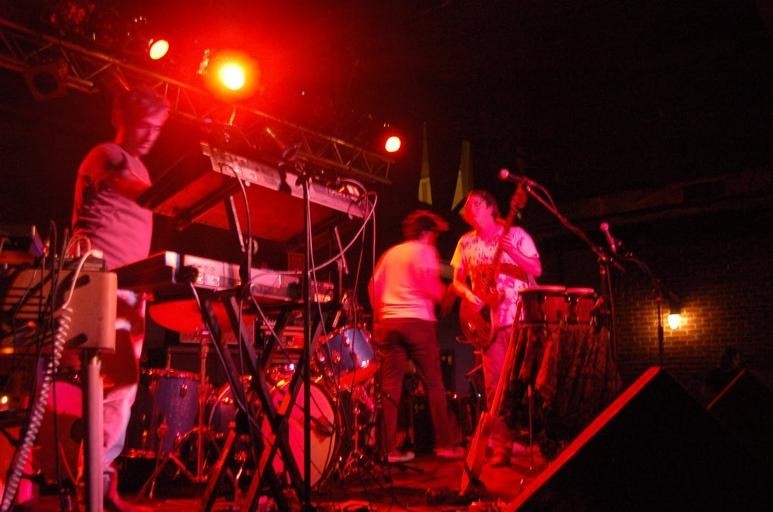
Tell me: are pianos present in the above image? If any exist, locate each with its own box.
[109,251,349,310]
[136,143,374,252]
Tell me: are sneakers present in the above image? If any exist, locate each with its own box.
[379,448,416,465]
[432,445,469,460]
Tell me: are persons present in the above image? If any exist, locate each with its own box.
[448,187,541,452]
[366,211,467,464]
[64,89,169,512]
[700,346,751,439]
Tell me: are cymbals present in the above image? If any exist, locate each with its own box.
[149,299,258,343]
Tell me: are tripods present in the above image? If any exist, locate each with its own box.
[137,342,243,504]
[322,392,408,512]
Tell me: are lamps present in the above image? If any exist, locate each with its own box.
[127,14,171,63]
[667,306,681,329]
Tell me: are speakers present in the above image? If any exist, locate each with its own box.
[501,367,702,512]
[709,365,768,452]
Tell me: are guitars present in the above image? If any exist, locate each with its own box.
[460,183,528,347]
[459,299,524,498]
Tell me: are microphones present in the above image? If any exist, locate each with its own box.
[600,221,616,252]
[499,169,533,186]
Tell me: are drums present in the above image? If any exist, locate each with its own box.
[201,363,349,498]
[121,366,201,459]
[519,285,568,327]
[565,285,596,328]
[310,321,381,397]
